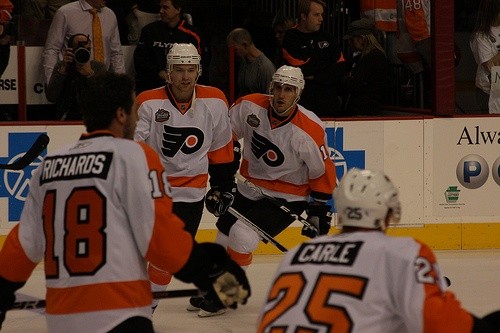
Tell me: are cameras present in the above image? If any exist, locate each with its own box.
[71,41,91,65]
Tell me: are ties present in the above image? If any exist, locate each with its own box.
[89,8,104,63]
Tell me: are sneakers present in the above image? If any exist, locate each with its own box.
[198,296,228,317]
[187,296,202,312]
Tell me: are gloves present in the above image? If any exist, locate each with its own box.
[189,242,251,311]
[0,292,16,329]
[473,310,500,333]
[301,203,332,237]
[206,181,237,218]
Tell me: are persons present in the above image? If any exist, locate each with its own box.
[272,0,432,116]
[46,34,117,121]
[127,0,160,45]
[186,65,336,318]
[0,0,71,76]
[41,0,127,85]
[470,0,500,112]
[133,0,208,94]
[258,168,500,333]
[133,43,234,315]
[227,29,276,95]
[0,74,251,333]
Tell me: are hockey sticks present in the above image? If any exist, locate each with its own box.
[228,206,288,254]
[235,172,318,235]
[6,284,243,311]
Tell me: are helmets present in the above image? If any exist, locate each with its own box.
[270,65,304,98]
[166,43,201,71]
[332,167,398,229]
